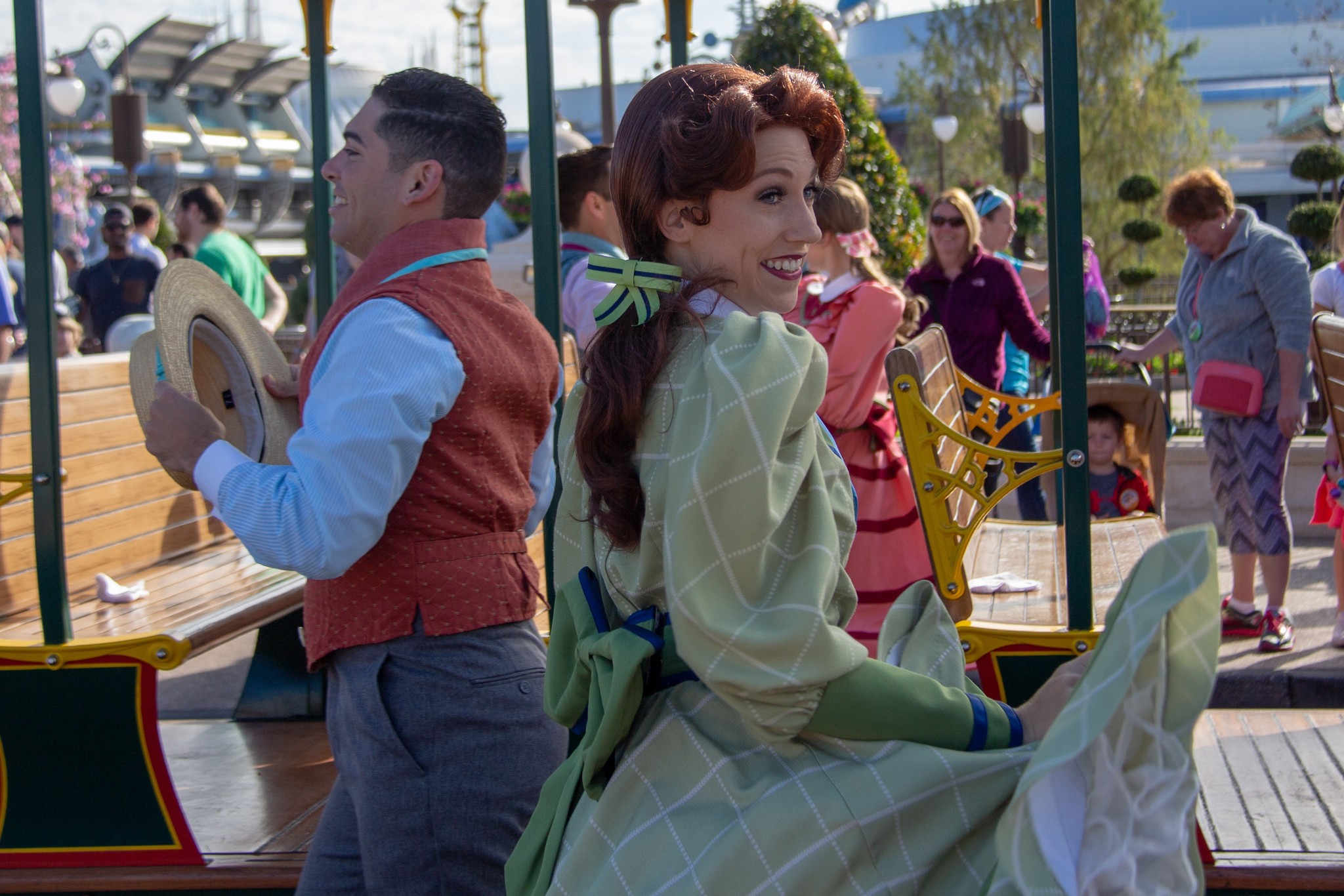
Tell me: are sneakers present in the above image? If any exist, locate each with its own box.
[1258,608,1295,651]
[1220,595,1264,636]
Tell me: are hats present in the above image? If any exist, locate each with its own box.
[128,258,301,492]
[102,201,134,225]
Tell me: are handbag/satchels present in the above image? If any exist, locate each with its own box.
[1190,361,1264,416]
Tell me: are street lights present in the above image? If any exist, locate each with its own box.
[45,21,144,219]
[1010,59,1045,263]
[931,81,960,192]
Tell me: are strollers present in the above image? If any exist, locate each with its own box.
[1037,342,1178,523]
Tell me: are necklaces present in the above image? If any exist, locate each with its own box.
[1187,273,1201,342]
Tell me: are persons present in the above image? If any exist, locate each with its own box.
[1110,167,1318,650]
[502,63,1220,896]
[488,119,593,315]
[902,187,1051,445]
[1309,415,1344,649]
[1088,403,1156,521]
[482,200,519,253]
[782,176,935,661]
[558,145,627,373]
[0,181,288,363]
[1311,200,1344,317]
[147,66,569,896]
[971,186,1089,521]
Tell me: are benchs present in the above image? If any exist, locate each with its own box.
[0,347,326,870]
[879,318,1176,705]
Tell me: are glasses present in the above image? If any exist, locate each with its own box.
[103,219,131,231]
[931,215,966,227]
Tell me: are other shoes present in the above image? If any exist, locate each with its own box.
[1332,611,1344,648]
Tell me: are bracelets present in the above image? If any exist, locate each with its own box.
[1322,460,1338,471]
